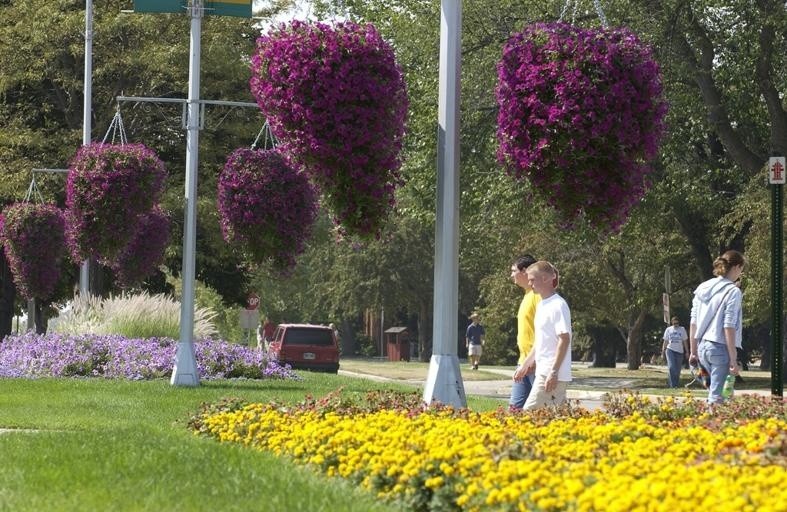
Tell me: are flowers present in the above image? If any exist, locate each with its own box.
[1,142,174,303]
[248,17,411,250]
[217,146,323,272]
[495,19,669,242]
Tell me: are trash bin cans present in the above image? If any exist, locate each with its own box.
[588,319,614,368]
[384,327,412,361]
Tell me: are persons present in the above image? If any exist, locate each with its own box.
[509,253,543,409]
[660,315,691,388]
[512,260,574,409]
[464,313,488,371]
[258,315,340,356]
[687,250,747,402]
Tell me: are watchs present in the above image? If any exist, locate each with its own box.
[550,369,559,378]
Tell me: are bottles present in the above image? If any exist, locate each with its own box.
[720,373,736,399]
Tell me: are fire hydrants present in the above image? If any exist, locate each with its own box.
[771,159,785,180]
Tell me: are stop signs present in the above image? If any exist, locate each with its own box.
[663,293,669,324]
[244,292,259,311]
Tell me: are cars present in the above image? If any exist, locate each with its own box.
[268,323,341,375]
